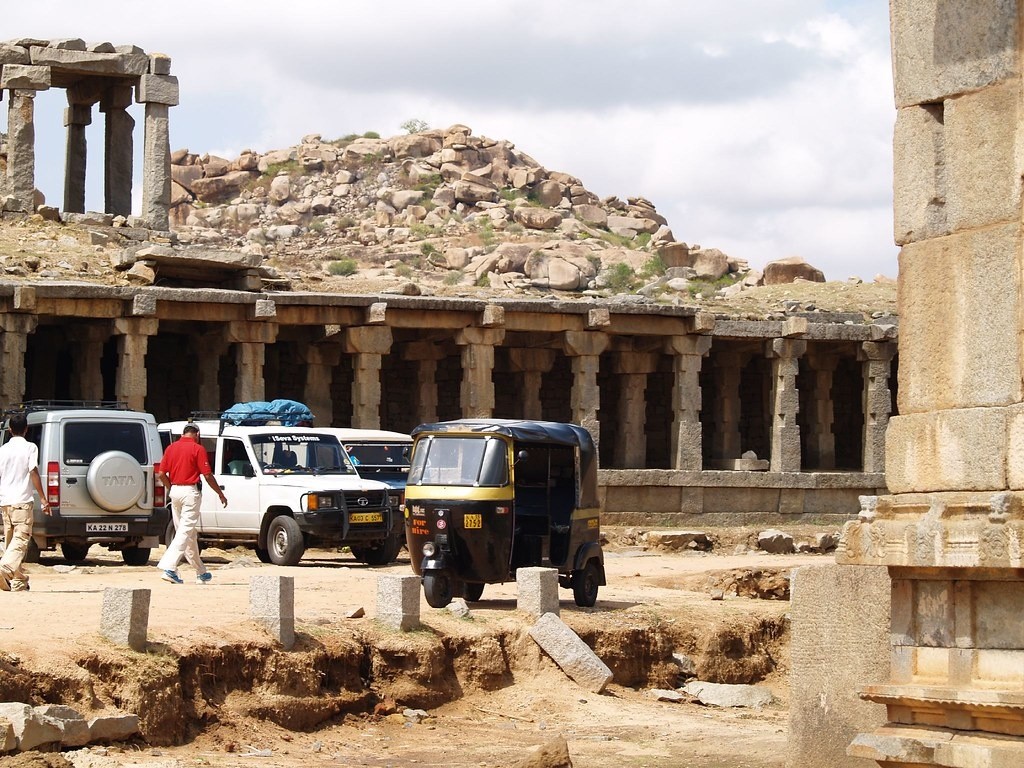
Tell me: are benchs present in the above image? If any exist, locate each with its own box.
[513,503,573,564]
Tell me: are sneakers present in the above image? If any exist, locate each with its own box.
[197,572,212,585]
[162,570,183,584]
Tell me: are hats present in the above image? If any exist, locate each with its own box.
[183,423,202,445]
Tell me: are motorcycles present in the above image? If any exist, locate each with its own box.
[401,418,607,608]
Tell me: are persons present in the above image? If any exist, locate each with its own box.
[156,422,228,585]
[0,413,50,591]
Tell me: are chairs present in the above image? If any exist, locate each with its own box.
[277,450,297,466]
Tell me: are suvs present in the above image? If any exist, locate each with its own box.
[257,427,464,564]
[156,410,394,566]
[0,398,173,567]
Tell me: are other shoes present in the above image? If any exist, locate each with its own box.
[0,573,11,591]
[26,584,30,590]
